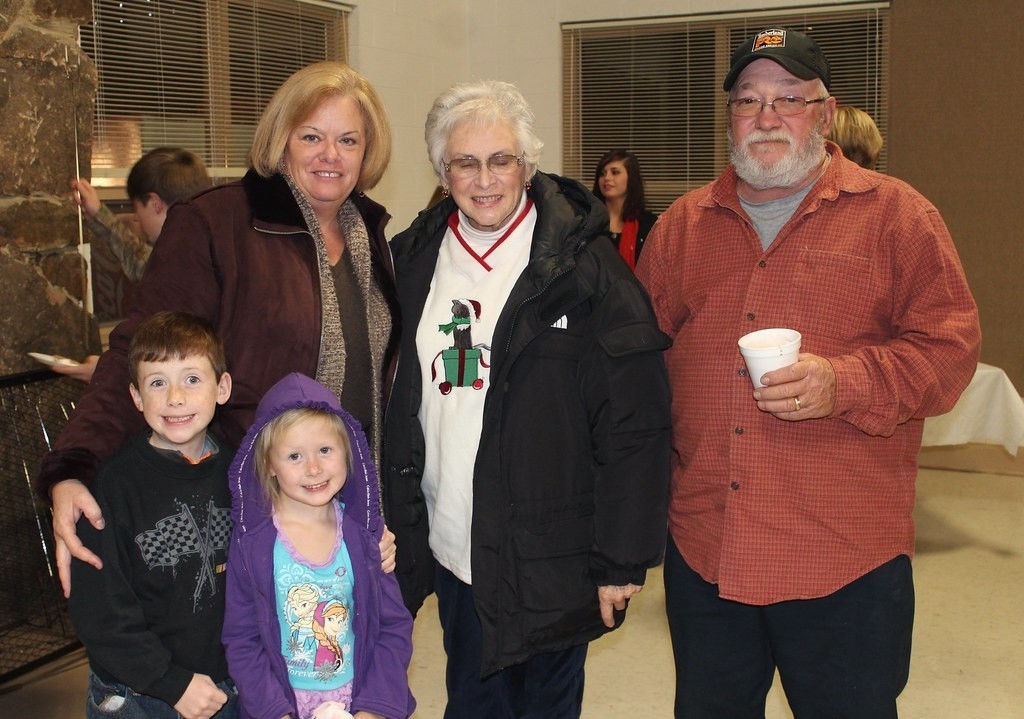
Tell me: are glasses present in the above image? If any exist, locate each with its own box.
[442,151,525,178]
[727,94,824,117]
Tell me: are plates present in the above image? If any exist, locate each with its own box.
[28,352,81,367]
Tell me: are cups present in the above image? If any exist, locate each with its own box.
[738,329,802,389]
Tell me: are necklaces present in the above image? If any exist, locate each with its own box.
[609,227,622,238]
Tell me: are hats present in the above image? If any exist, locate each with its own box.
[723,26,831,91]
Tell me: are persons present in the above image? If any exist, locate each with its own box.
[70,95,890,289]
[63,309,240,719]
[218,369,416,719]
[46,61,404,626]
[634,23,982,719]
[382,78,675,719]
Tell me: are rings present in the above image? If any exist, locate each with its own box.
[794,396,801,411]
